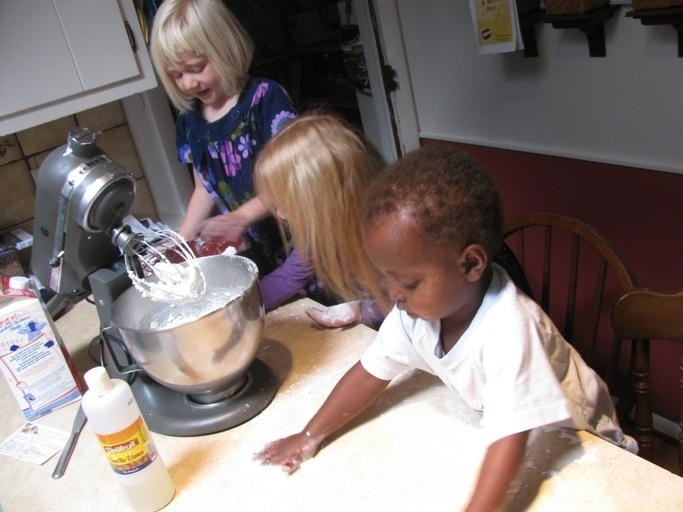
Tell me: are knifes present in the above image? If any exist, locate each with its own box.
[50,403,87,480]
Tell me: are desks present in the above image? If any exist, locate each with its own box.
[0,291,683,512]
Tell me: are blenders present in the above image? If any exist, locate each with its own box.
[30,127,278,436]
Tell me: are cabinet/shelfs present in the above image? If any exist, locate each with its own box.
[0,0,159,139]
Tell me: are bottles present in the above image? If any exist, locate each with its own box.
[81,365,176,512]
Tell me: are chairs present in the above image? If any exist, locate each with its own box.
[611,289,682,476]
[499,211,637,431]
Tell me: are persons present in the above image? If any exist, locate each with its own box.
[253,145,640,512]
[248,112,394,330]
[148,0,332,304]
